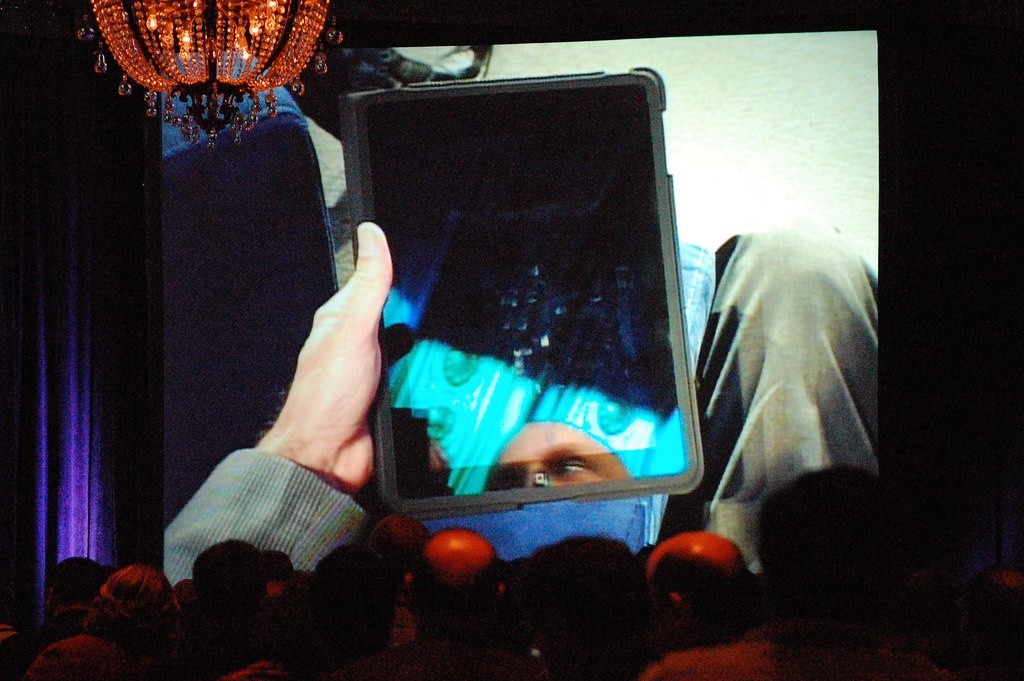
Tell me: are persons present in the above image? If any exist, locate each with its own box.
[1,467,1023,681]
[283,44,493,142]
[164,222,877,587]
[484,420,633,492]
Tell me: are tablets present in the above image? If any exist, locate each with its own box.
[355,73,696,510]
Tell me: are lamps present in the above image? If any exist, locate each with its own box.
[77,0,342,143]
